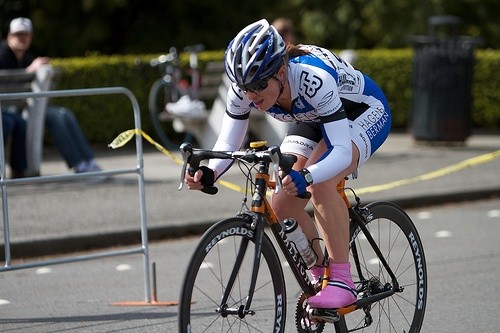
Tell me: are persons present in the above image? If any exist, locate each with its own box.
[273,17,296,43]
[185,18,392,330]
[1,111,28,179]
[0,18,115,180]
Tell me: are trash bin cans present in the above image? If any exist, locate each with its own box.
[410,37,478,142]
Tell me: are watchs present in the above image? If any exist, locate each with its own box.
[300,168,313,187]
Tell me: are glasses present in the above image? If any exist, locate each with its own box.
[237,71,278,94]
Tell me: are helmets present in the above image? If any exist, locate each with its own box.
[225,19,286,85]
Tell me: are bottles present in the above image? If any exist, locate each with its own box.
[281,218,316,270]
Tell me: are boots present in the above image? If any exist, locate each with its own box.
[310,266,324,280]
[307,261,358,308]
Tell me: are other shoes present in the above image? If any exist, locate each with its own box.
[76,165,111,181]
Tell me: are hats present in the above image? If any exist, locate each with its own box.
[10,18,33,34]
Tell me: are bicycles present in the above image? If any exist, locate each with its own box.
[177,141,429,332]
[133,44,203,152]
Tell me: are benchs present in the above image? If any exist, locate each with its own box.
[0,65,56,177]
[176,50,281,162]
